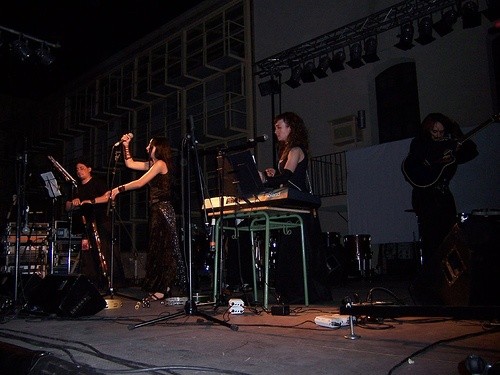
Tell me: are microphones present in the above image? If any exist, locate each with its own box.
[248,134,269,142]
[22,205,30,234]
[209,218,216,251]
[113,132,134,146]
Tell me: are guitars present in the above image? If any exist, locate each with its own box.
[399,114,499,191]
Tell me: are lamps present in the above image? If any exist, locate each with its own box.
[11,41,56,67]
[346,40,365,70]
[362,34,381,64]
[461,0,482,29]
[285,62,302,91]
[415,17,438,46]
[328,46,346,72]
[393,21,418,51]
[432,9,459,38]
[313,51,330,80]
[358,110,366,129]
[299,57,317,84]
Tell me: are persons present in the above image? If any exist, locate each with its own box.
[401,113,480,304]
[257,112,327,304]
[109,132,188,302]
[64,159,119,295]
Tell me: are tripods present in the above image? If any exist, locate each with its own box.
[128,117,238,332]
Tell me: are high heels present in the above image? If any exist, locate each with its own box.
[151,286,171,301]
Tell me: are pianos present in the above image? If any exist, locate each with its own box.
[199,187,323,312]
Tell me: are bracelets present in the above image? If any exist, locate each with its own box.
[117,185,126,193]
[89,198,95,204]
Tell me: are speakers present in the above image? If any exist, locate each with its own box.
[0,274,106,317]
[421,223,500,304]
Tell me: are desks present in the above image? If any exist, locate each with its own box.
[207,206,313,310]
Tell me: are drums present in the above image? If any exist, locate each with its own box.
[320,231,343,285]
[342,233,372,282]
